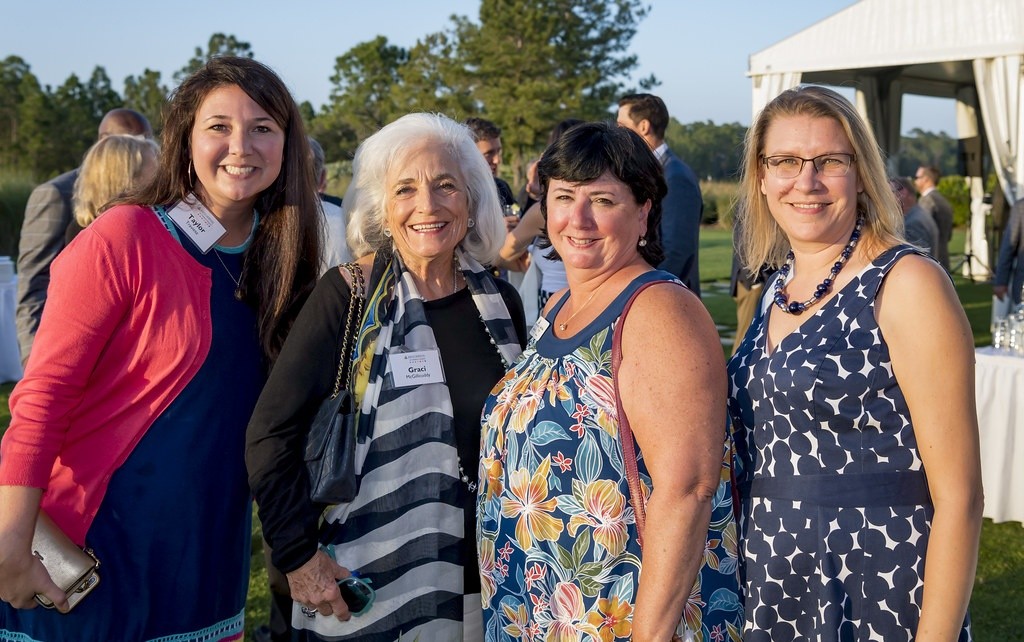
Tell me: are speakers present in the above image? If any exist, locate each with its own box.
[957,136,990,177]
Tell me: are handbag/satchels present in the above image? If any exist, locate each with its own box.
[519,262,544,327]
[304,264,367,502]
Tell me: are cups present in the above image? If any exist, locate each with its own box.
[993,314,1024,355]
[503,204,522,219]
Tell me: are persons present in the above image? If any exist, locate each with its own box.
[616,93,703,299]
[65,134,162,245]
[914,165,953,270]
[244,111,526,642]
[890,177,938,260]
[727,85,985,642]
[16,109,154,372]
[730,201,778,354]
[475,124,743,642]
[309,138,357,277]
[992,197,1024,303]
[0,57,321,642]
[464,117,586,312]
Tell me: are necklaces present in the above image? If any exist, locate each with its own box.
[421,255,509,492]
[774,210,865,315]
[213,247,247,299]
[560,285,602,331]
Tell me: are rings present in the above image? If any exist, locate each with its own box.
[301,606,318,618]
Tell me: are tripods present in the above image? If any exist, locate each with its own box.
[951,176,997,284]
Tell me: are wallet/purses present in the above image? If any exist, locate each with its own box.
[27,507,99,608]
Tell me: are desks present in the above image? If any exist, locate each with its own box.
[0,274,24,383]
[974,347,1024,528]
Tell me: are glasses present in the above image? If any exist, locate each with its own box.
[763,152,856,179]
[320,543,376,618]
